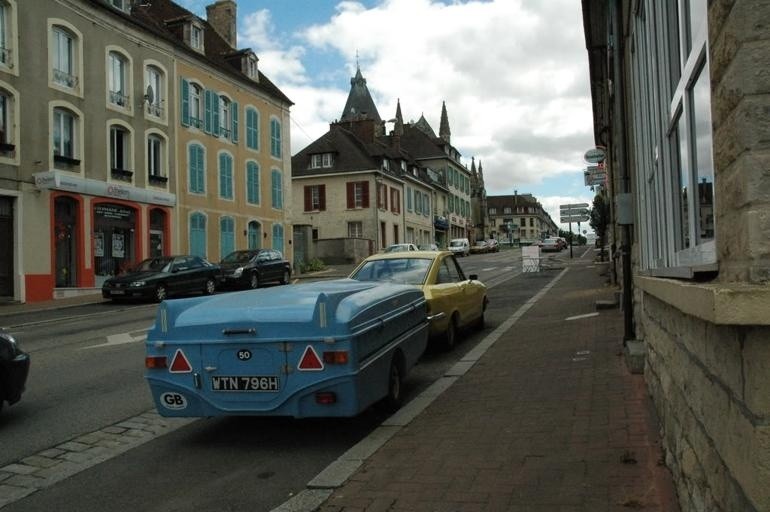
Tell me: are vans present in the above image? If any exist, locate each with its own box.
[448,238,471,256]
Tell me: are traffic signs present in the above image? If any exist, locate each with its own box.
[559,203,589,223]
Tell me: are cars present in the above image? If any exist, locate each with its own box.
[472,240,487,253]
[541,237,567,252]
[349,243,489,347]
[219,249,292,289]
[0,327,29,411]
[384,243,419,253]
[487,239,500,252]
[102,256,223,302]
